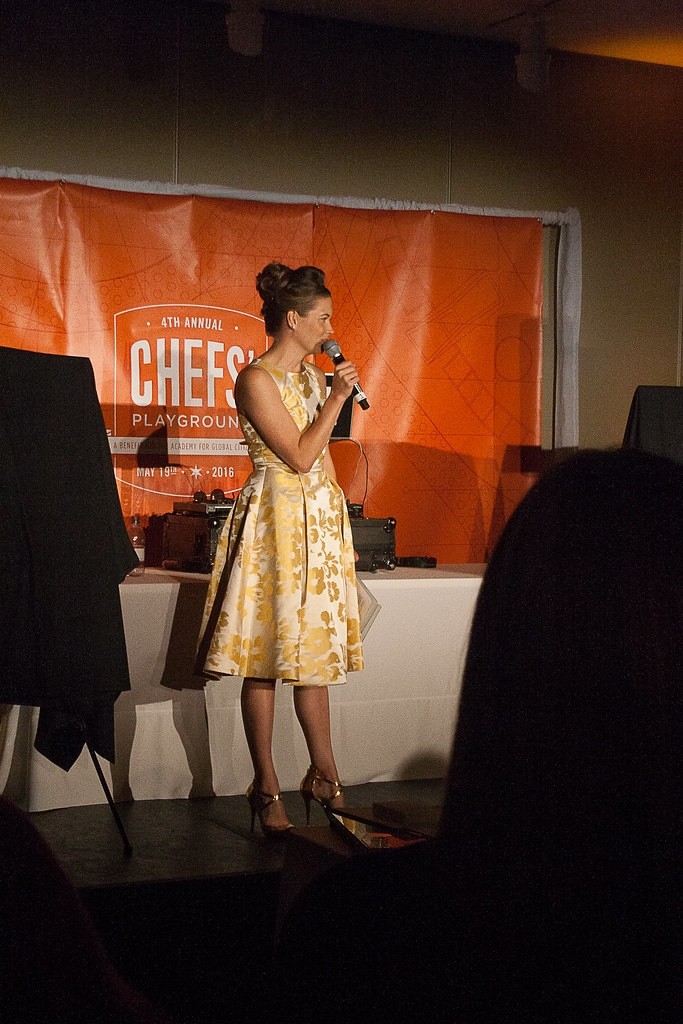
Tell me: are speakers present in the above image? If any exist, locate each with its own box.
[621,385,683,468]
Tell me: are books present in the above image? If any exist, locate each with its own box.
[354,572,380,645]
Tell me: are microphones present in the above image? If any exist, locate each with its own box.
[323,339,369,411]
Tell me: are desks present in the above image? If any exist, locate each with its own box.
[0,562,486,813]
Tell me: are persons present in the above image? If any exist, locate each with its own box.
[194,264,364,841]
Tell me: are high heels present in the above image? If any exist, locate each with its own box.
[300,765,346,826]
[246,780,299,837]
